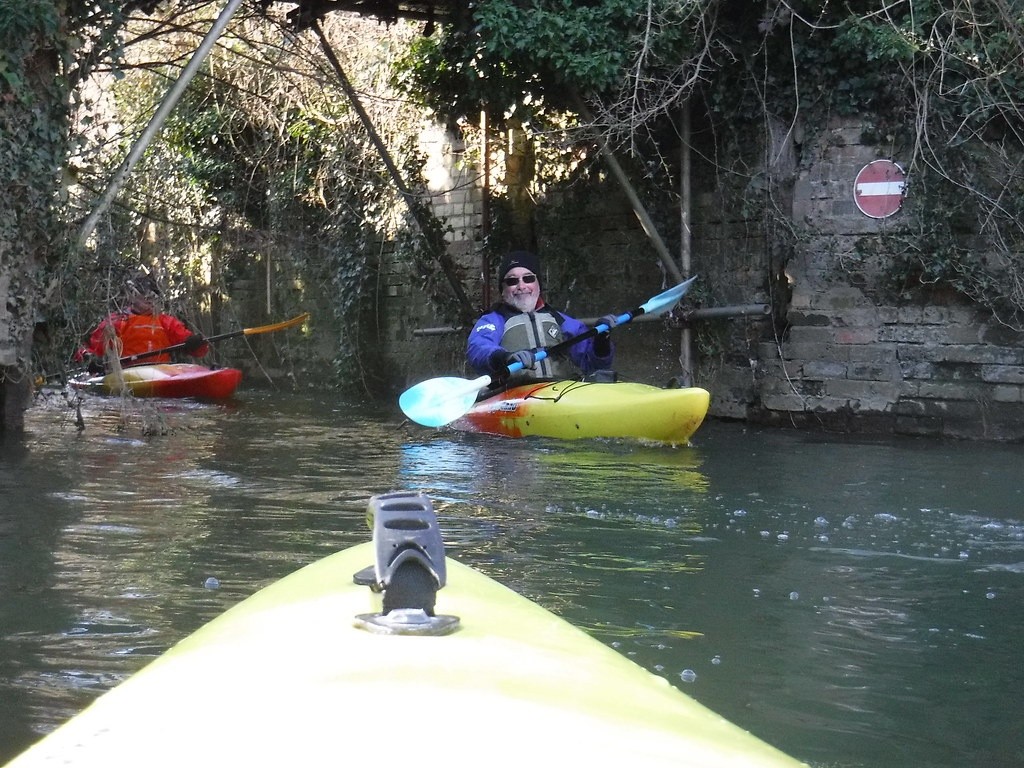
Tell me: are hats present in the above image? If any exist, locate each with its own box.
[499,251,538,294]
[130,275,159,294]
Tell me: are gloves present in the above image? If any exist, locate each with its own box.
[490,348,545,370]
[87,358,105,376]
[594,314,617,358]
[184,334,206,350]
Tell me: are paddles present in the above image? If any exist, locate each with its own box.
[399,273,697,428]
[35,312,313,384]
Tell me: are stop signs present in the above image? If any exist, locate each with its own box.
[853,159,908,219]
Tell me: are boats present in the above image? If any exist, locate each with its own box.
[68,363,242,399]
[448,371,710,442]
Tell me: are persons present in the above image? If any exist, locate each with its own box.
[467,251,618,389]
[82,273,210,377]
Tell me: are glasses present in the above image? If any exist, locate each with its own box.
[502,274,537,286]
